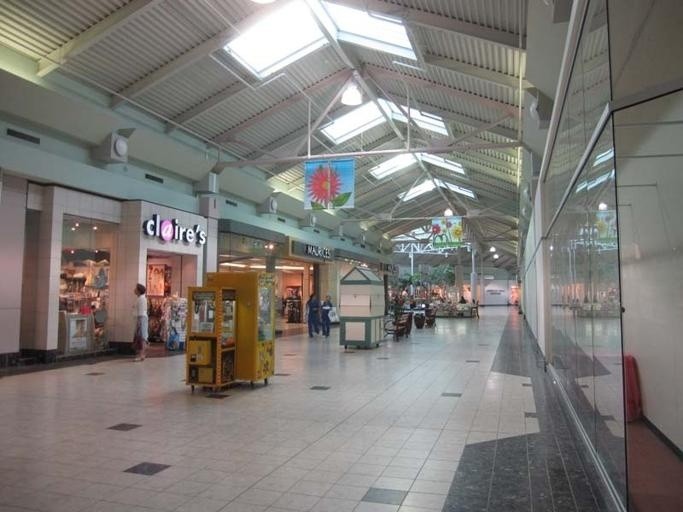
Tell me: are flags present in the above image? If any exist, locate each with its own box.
[431,216,464,249]
[303,159,354,211]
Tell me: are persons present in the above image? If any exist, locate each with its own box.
[305,291,321,337]
[456,295,465,315]
[130,281,149,361]
[319,294,334,337]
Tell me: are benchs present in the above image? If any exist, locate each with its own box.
[383,320,407,342]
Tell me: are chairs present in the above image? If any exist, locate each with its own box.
[393,311,413,338]
